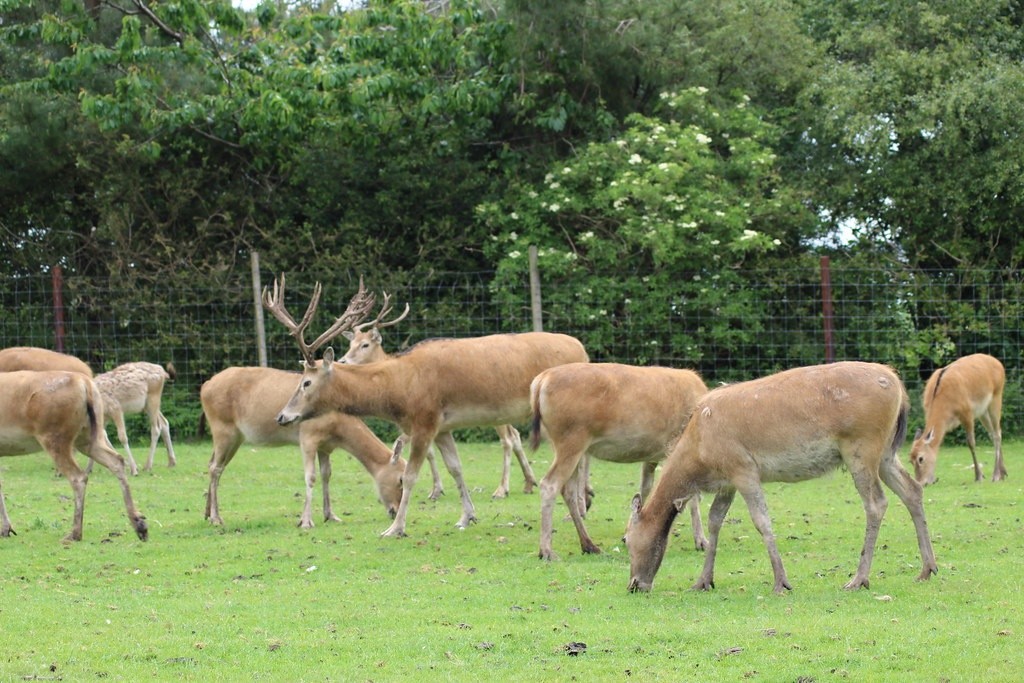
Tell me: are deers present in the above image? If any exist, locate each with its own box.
[262,269,598,539]
[622,361,939,597]
[336,287,539,500]
[908,353,1007,486]
[199,364,408,526]
[525,361,711,561]
[85,361,175,476]
[0,345,149,545]
